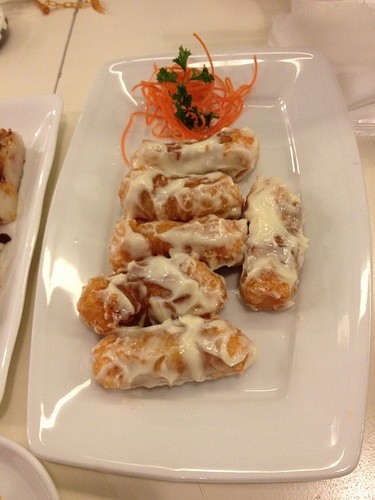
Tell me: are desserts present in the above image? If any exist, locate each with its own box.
[75,127,310,391]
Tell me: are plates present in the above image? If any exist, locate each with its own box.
[0,94,63,405]
[26,45,374,484]
[0,435,58,500]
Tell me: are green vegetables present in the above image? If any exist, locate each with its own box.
[157,45,219,130]
